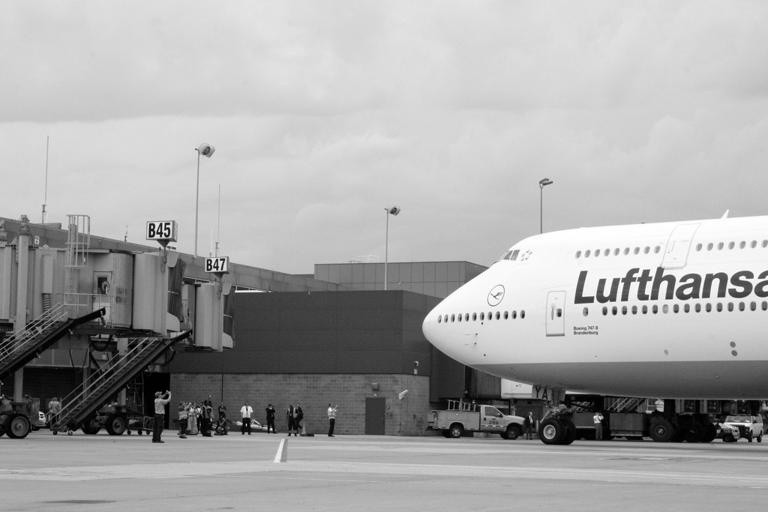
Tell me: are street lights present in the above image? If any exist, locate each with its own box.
[381,204,402,291]
[537,175,554,233]
[192,140,214,255]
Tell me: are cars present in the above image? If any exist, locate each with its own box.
[31,410,47,431]
[714,420,741,443]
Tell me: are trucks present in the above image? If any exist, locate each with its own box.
[427,403,526,441]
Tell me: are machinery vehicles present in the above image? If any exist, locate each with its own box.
[45,326,193,437]
[0,293,105,441]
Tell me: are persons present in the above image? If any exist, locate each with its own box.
[178,400,227,438]
[287,405,297,436]
[295,404,303,433]
[49,397,59,436]
[524,411,535,440]
[152,391,171,443]
[240,403,253,435]
[328,403,337,437]
[266,404,275,433]
[593,412,604,440]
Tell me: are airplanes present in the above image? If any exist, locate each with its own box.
[421,210,766,446]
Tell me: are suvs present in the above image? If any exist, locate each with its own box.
[722,414,765,442]
